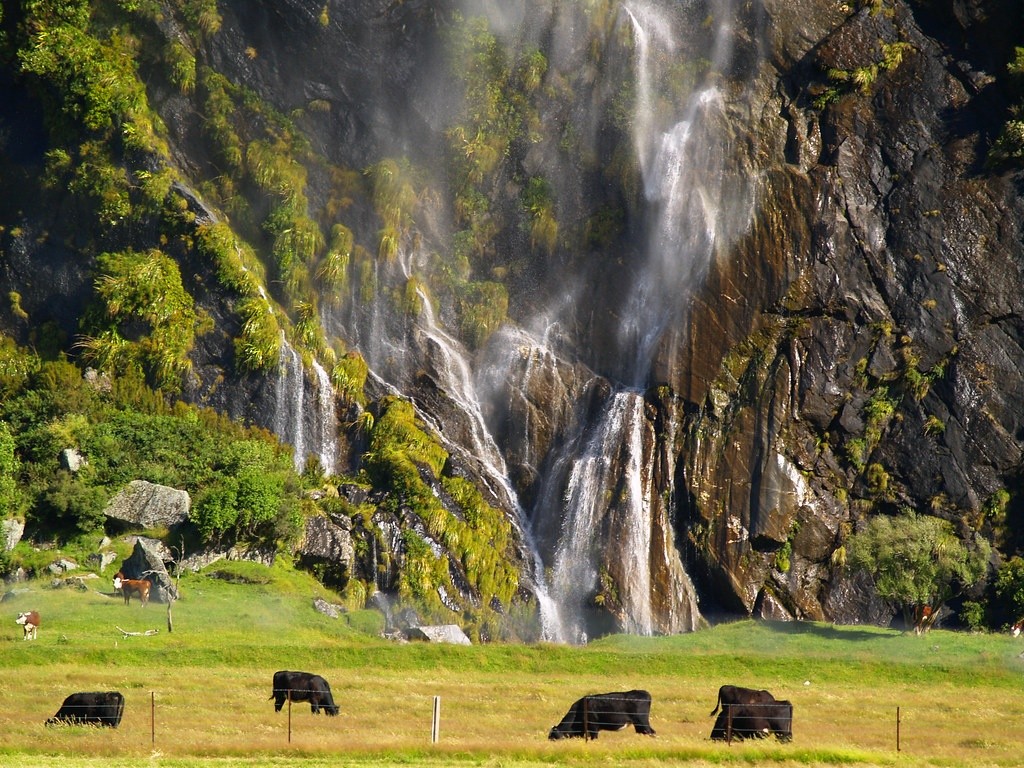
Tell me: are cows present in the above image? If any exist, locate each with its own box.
[267,670,340,716]
[113,573,151,608]
[549,689,656,741]
[44,690,125,729]
[709,685,793,744]
[15,611,41,641]
